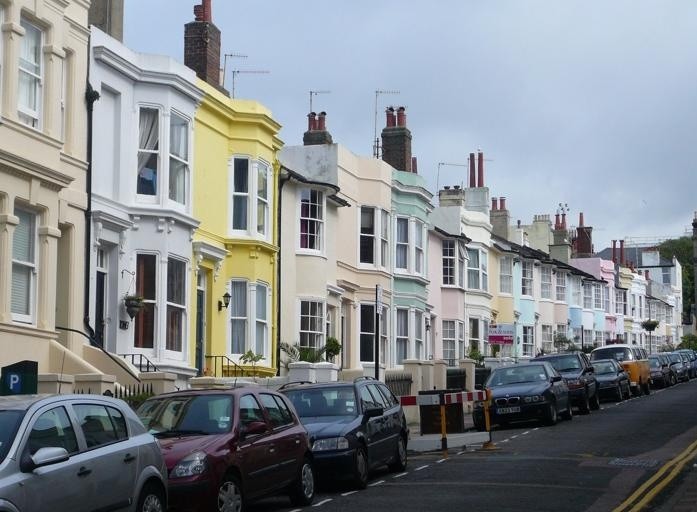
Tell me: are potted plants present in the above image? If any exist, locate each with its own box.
[123,294,144,322]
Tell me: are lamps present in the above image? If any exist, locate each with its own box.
[218,292,231,310]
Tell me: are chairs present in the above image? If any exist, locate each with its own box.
[29,415,105,462]
[308,396,348,417]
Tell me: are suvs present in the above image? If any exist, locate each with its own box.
[274,375,410,491]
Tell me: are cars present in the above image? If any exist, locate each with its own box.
[473,344,697,431]
[0,393,169,512]
[134,385,316,512]
